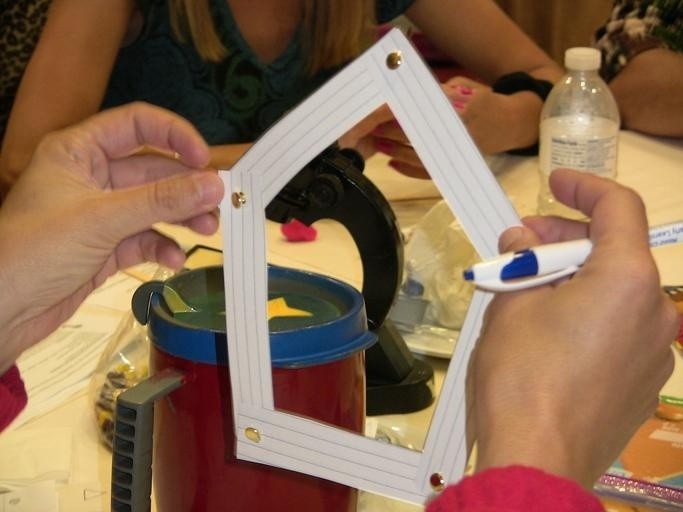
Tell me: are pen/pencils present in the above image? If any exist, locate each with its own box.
[462,222,683,292]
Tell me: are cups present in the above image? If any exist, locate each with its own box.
[115,259,368,506]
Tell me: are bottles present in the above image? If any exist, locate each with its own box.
[90,255,183,450]
[539,47,622,220]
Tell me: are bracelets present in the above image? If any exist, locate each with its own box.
[489,71,560,159]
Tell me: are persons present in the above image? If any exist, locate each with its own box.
[0,0,569,204]
[591,0,682,139]
